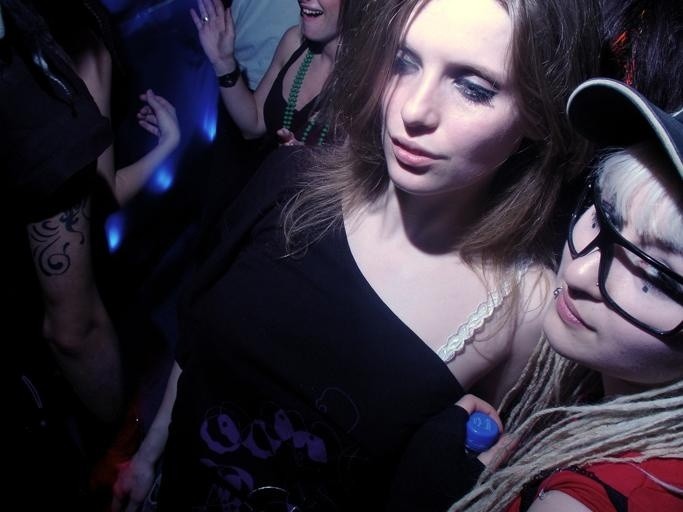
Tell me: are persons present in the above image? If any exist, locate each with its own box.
[106,0,621,511]
[438,77,682,512]
[0,0,377,511]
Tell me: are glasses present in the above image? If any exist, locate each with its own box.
[566,178,683,337]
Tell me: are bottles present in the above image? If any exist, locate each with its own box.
[461,409,497,452]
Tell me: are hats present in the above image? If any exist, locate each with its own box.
[568,77,683,176]
